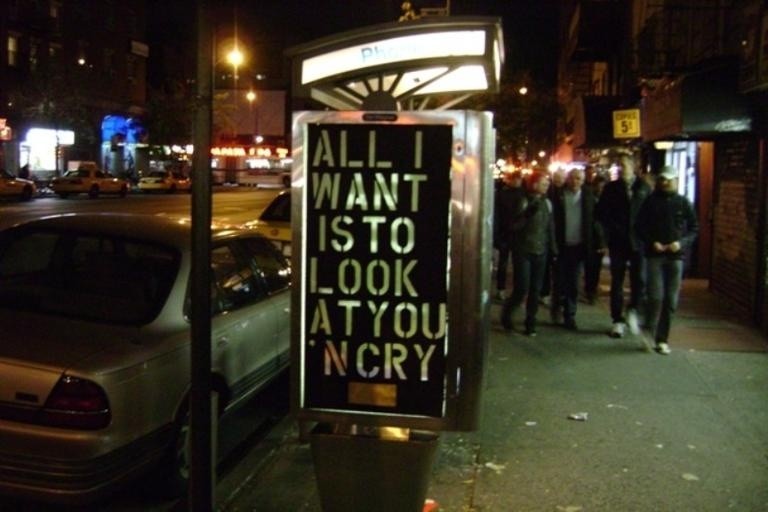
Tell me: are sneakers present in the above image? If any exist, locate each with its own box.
[498,293,675,356]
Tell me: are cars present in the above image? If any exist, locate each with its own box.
[138,172,190,194]
[242,189,292,268]
[49,166,128,199]
[1,169,36,202]
[1,213,294,497]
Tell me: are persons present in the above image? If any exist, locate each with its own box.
[19,164,31,179]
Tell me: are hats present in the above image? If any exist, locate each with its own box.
[656,165,680,180]
